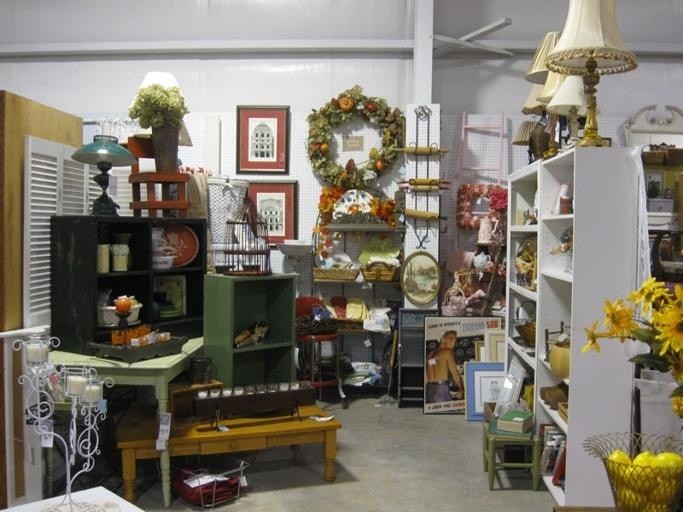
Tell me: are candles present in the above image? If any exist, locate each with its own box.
[81,386,100,403]
[26,343,46,363]
[67,375,87,395]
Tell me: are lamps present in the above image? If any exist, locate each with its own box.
[278,239,313,297]
[546,0,637,146]
[578,275,682,419]
[546,73,601,147]
[537,71,570,154]
[524,31,563,148]
[513,120,541,163]
[72,136,139,216]
[523,83,549,161]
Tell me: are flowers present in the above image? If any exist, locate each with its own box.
[313,187,397,261]
[309,88,405,188]
[456,185,509,230]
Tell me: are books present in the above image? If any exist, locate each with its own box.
[497,411,534,434]
[538,424,566,487]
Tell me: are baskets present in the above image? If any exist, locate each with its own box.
[441,286,467,317]
[206,177,250,244]
[514,300,536,347]
[359,260,401,282]
[313,267,360,280]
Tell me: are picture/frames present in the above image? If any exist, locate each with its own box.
[645,171,665,199]
[649,234,668,239]
[650,239,671,260]
[154,275,187,316]
[464,362,505,421]
[486,328,506,363]
[476,340,485,360]
[424,317,502,414]
[248,182,298,247]
[494,375,514,415]
[494,356,526,417]
[234,105,289,174]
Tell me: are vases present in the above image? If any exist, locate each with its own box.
[630,361,662,459]
[637,370,683,455]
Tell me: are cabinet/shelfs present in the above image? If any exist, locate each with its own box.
[51,215,208,364]
[204,271,298,417]
[622,108,683,283]
[313,187,404,391]
[507,148,638,512]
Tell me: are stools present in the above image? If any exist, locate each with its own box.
[299,334,341,402]
[483,423,540,491]
[129,173,192,217]
[155,377,222,419]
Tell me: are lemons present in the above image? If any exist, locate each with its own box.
[652,452,683,477]
[609,447,632,476]
[633,452,655,467]
[615,470,678,512]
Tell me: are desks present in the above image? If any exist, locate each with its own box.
[0,485,146,512]
[47,336,205,507]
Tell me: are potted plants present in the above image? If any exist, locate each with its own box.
[127,85,189,173]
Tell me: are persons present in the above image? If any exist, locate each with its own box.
[426,330,464,402]
